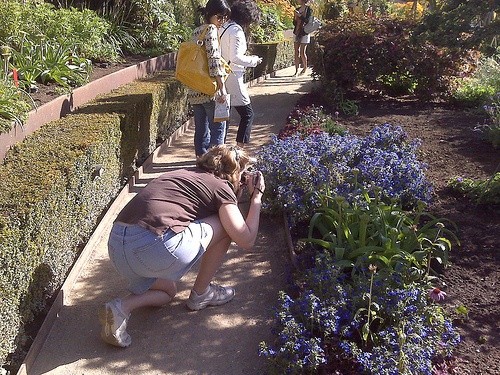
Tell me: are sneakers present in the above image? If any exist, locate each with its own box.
[100,298,131,347]
[187,282,236,311]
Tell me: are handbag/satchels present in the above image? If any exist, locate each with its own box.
[304,17,323,34]
[175,26,232,95]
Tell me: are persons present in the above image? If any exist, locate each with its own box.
[99,144,265,348]
[293,0,311,77]
[218,0,262,150]
[188,0,231,157]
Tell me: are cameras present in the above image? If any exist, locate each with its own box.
[241,170,258,185]
[294,10,299,14]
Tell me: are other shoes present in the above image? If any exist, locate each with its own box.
[234,148,257,163]
[293,70,298,76]
[301,68,306,74]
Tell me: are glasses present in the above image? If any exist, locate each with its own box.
[214,14,228,24]
